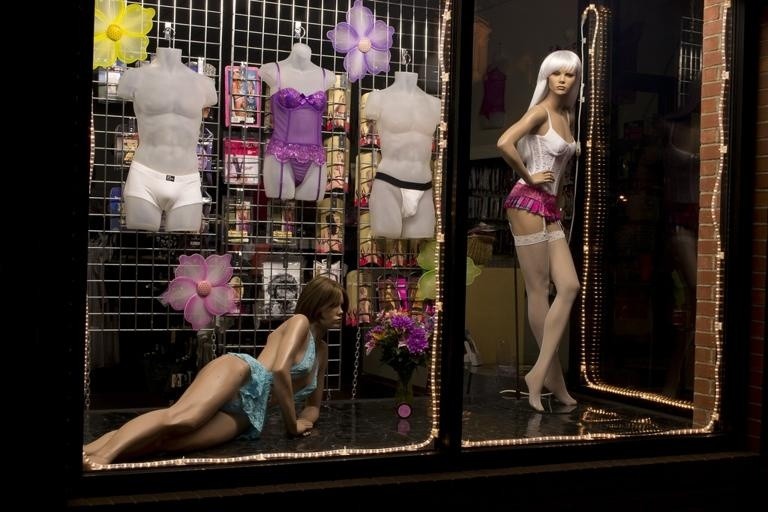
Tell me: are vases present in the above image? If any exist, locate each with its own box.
[390,367,417,418]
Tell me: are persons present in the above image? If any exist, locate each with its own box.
[83,276,349,465]
[366,72,441,238]
[259,43,338,204]
[496,50,582,413]
[117,47,217,232]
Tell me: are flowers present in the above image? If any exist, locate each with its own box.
[360,303,436,388]
